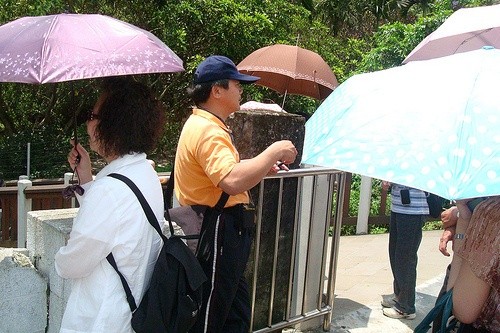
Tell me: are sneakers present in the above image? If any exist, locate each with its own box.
[382,298,399,307]
[383,306,416,319]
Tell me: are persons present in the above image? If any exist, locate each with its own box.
[55,77,167,333]
[172,56,298,333]
[381,180,430,319]
[438,196,500,333]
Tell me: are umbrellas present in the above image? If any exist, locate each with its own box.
[402,4,500,63]
[299,46,500,203]
[0,13,185,164]
[235,35,338,111]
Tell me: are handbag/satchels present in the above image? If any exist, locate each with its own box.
[162,204,220,263]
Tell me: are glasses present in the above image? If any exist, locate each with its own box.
[209,81,243,90]
[87,110,101,121]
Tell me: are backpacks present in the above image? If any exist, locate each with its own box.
[426,192,442,219]
[102,171,207,333]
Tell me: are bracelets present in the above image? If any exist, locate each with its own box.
[454,234,464,239]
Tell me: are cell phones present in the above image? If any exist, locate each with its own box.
[400,189,411,204]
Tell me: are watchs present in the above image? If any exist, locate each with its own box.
[457,212,459,217]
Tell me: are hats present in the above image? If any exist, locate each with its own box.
[194,55,261,84]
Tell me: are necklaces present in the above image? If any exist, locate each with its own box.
[197,104,233,144]
